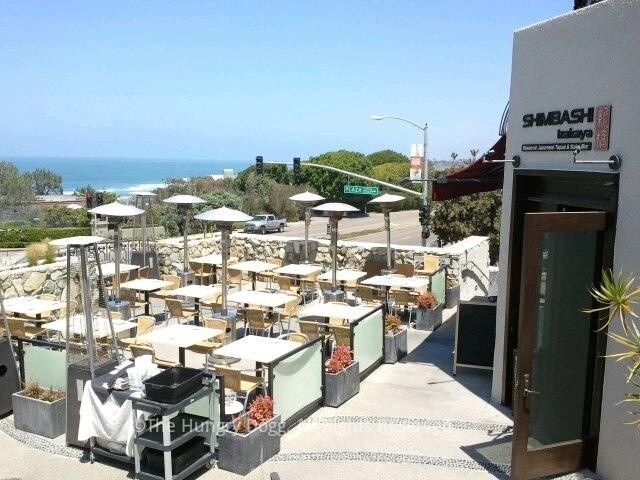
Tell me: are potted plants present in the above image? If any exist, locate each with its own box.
[10,381,65,440]
[417,291,443,331]
[446,280,461,309]
[221,395,282,476]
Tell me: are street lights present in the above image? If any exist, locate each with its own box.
[367,114,430,209]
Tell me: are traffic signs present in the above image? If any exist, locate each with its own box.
[344,185,364,194]
[363,187,379,196]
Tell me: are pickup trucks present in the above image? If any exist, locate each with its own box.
[244,212,288,235]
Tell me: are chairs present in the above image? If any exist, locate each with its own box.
[0,250,421,410]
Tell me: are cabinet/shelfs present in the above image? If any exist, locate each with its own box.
[453,296,498,379]
[88,367,220,480]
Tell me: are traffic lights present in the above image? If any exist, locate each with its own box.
[254,155,263,174]
[293,157,300,177]
[418,203,430,226]
